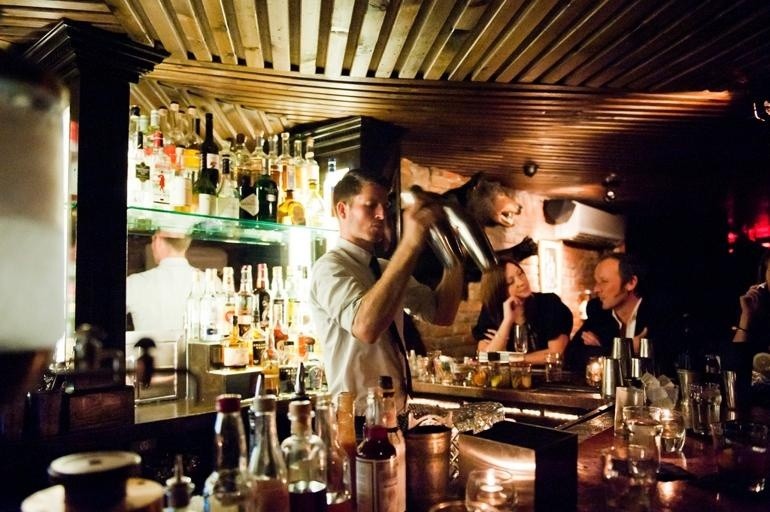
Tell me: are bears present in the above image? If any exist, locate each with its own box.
[410,170,539,301]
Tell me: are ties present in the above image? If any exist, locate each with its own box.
[370,256,416,402]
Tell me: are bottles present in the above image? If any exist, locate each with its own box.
[167,376,519,512]
[404,325,564,390]
[128,102,327,228]
[321,155,343,229]
[584,337,742,482]
[182,262,324,396]
[399,186,498,278]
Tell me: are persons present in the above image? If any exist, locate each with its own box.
[473,258,572,362]
[561,252,688,376]
[124,229,240,340]
[309,167,463,435]
[718,252,770,407]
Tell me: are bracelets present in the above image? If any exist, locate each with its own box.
[734,323,750,333]
